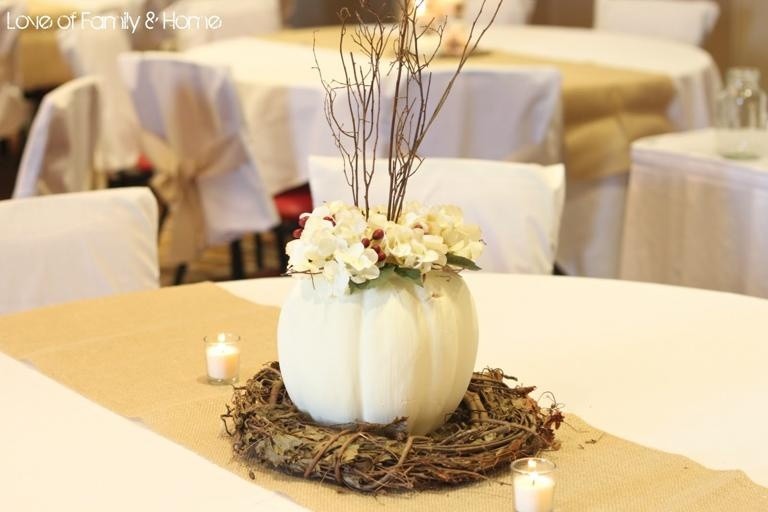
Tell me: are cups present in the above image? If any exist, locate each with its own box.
[510,457,558,512]
[203,333,244,385]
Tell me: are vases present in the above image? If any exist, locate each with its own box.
[278,270,479,436]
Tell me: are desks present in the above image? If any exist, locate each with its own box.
[0,268,768,511]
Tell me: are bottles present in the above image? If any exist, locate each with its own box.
[713,67,768,161]
